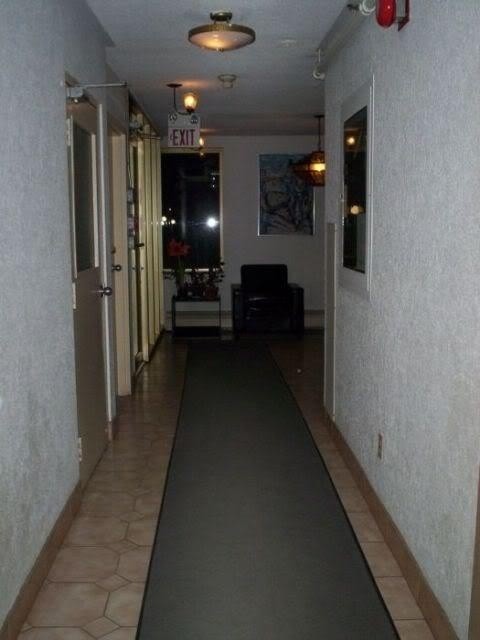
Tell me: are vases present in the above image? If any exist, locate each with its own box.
[175,282,188,301]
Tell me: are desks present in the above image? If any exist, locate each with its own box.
[170,295,223,344]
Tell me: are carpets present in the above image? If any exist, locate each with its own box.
[131,336,400,640]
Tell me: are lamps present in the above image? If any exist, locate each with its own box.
[187,10,257,53]
[165,81,200,118]
[293,113,327,188]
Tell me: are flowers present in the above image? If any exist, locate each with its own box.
[161,239,192,288]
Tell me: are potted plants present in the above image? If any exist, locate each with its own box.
[186,253,226,301]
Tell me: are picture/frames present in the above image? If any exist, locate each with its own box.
[255,150,315,239]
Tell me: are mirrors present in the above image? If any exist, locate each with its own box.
[334,73,376,305]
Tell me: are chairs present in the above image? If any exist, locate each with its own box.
[229,261,306,348]
